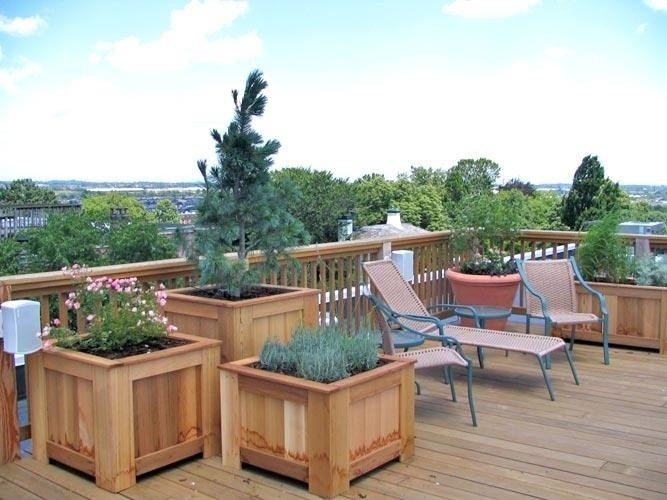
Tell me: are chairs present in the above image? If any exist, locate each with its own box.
[368,293,478,428]
[514,256,610,365]
[362,259,579,400]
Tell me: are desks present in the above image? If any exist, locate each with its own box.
[456,304,512,356]
[375,329,425,394]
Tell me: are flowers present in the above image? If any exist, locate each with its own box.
[36,260,179,351]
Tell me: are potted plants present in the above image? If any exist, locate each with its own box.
[444,192,523,333]
[215,302,418,497]
[153,69,322,363]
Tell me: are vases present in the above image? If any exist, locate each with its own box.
[26,329,224,493]
